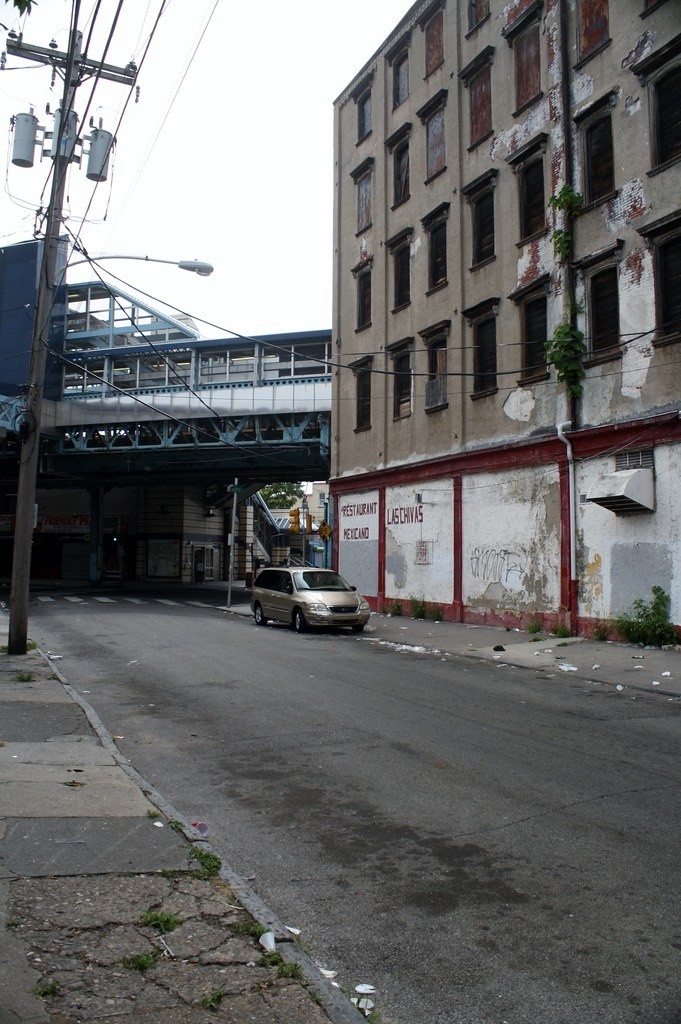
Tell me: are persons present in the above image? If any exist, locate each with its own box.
[254,559,260,578]
[196,560,205,584]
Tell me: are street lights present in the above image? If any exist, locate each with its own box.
[7,255,214,656]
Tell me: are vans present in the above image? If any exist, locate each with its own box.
[249,567,370,635]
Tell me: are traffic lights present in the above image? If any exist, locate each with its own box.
[288,508,300,533]
